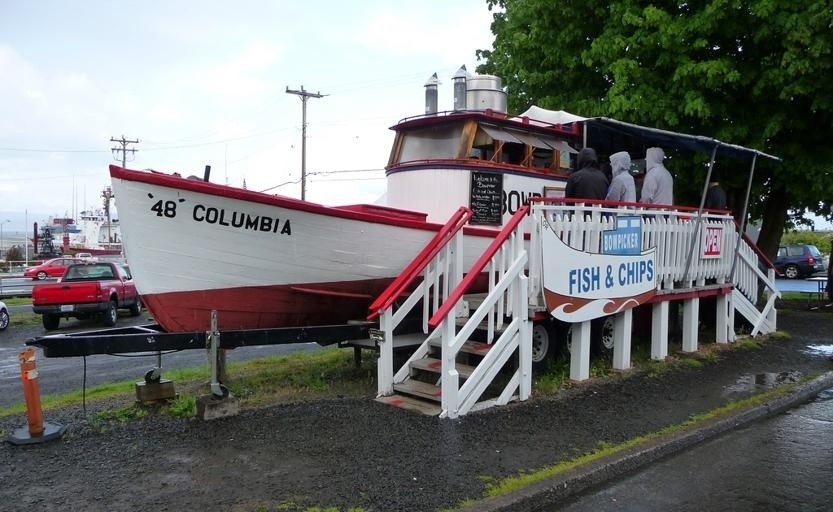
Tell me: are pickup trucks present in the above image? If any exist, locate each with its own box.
[74,253,98,264]
[31,260,142,332]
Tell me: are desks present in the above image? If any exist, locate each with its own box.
[806,277,829,310]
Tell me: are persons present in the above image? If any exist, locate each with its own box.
[705,174,726,209]
[565,148,673,218]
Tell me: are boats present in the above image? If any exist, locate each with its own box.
[26,136,145,257]
[103,61,595,339]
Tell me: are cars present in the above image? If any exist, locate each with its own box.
[774,243,825,280]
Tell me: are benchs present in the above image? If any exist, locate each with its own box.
[800,289,830,309]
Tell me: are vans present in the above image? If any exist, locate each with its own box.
[23,257,84,280]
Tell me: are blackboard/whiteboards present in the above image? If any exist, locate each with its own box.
[468,169,503,226]
